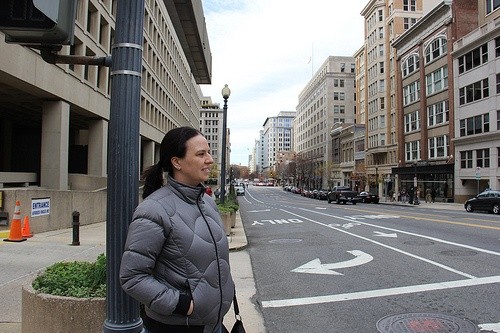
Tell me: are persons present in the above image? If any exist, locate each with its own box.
[392,185,436,205]
[119,127,236,333]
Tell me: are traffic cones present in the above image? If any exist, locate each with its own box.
[21,215,34,237]
[3,202,27,242]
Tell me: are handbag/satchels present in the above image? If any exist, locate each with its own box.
[221,316,245,333]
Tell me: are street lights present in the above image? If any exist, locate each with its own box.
[220,83,231,204]
[375,163,378,196]
[413,156,420,205]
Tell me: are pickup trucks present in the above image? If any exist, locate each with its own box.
[326,185,359,205]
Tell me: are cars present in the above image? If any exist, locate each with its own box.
[358,191,379,204]
[204,178,246,198]
[464,190,500,215]
[253,180,331,200]
